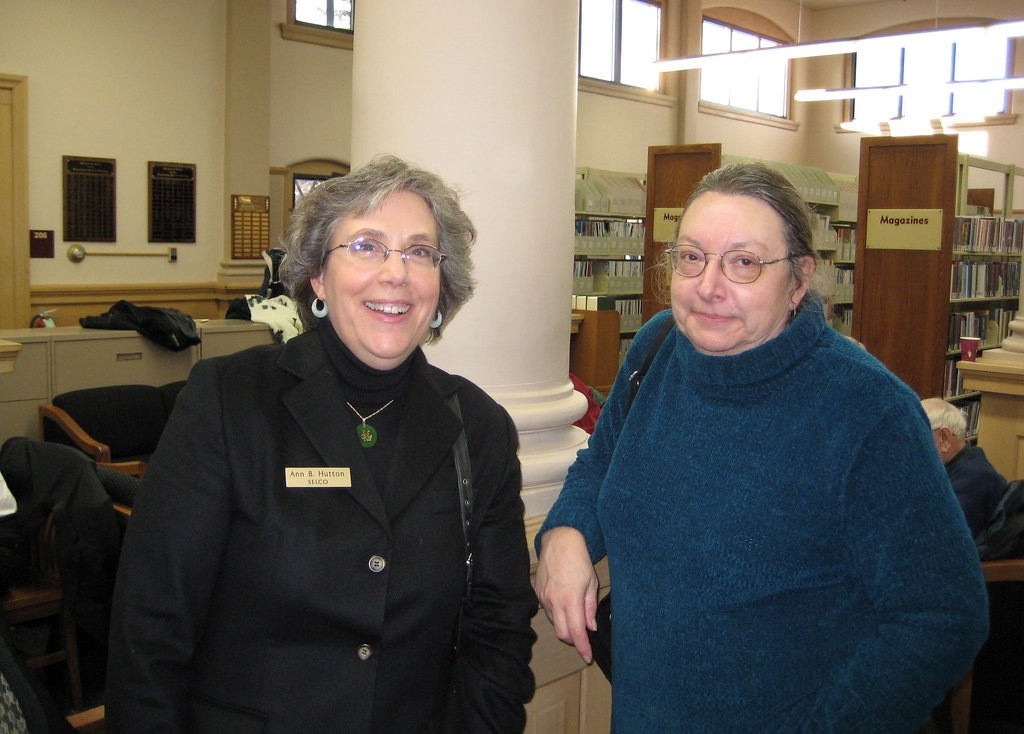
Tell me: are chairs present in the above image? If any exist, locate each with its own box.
[950,561,1024,734]
[0,436,96,710]
[37,381,182,524]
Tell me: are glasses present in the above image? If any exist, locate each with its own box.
[324,238,448,272]
[665,244,794,285]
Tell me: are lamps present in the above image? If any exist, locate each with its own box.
[651,0,1024,101]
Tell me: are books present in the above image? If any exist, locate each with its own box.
[805,207,857,336]
[943,217,1024,443]
[573,217,646,314]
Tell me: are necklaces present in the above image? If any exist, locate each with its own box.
[344,397,395,447]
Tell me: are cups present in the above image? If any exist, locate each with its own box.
[960,337,981,362]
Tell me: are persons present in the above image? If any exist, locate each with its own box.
[107,156,535,733]
[538,161,988,732]
[914,396,1012,540]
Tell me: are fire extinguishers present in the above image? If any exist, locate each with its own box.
[29,308,60,328]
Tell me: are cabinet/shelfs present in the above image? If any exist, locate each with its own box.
[0,319,277,450]
[523,553,614,734]
[569,133,1024,448]
